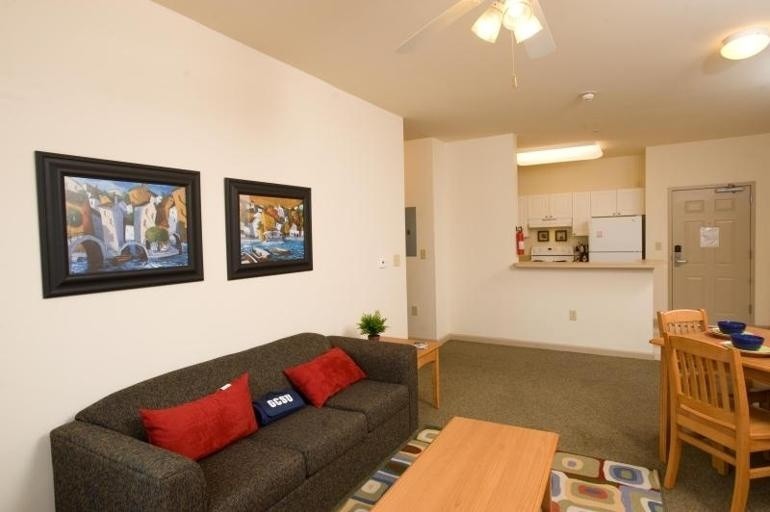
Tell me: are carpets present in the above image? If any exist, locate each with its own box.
[330,425,670,512]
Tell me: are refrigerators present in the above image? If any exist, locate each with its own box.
[588,216,643,262]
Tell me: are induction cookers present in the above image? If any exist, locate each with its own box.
[531,256,572,262]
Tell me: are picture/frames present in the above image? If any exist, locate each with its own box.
[555,230,567,241]
[224,176,316,282]
[538,230,549,242]
[33,148,206,300]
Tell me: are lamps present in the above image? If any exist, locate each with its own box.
[715,32,769,60]
[518,140,606,169]
[470,1,544,47]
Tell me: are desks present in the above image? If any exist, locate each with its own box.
[369,415,571,512]
[373,334,445,409]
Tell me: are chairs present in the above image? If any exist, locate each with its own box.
[658,307,707,463]
[660,335,770,511]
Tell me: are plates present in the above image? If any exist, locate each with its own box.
[720,340,770,355]
[706,326,754,338]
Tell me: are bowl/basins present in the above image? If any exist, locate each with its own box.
[731,334,766,348]
[717,319,746,335]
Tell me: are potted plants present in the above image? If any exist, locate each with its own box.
[357,307,389,343]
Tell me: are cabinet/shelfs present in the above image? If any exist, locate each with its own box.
[591,190,645,216]
[573,192,590,235]
[521,195,572,229]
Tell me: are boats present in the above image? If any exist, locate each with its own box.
[252,245,270,262]
[149,243,179,260]
[110,253,132,265]
[270,246,290,257]
[263,230,285,246]
[241,250,257,264]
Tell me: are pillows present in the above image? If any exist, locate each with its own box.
[136,367,262,462]
[284,345,366,410]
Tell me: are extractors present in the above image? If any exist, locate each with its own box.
[527,217,573,228]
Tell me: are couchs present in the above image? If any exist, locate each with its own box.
[51,333,419,512]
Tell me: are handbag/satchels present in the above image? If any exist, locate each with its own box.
[252,389,304,424]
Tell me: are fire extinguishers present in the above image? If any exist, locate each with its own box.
[516,227,526,256]
